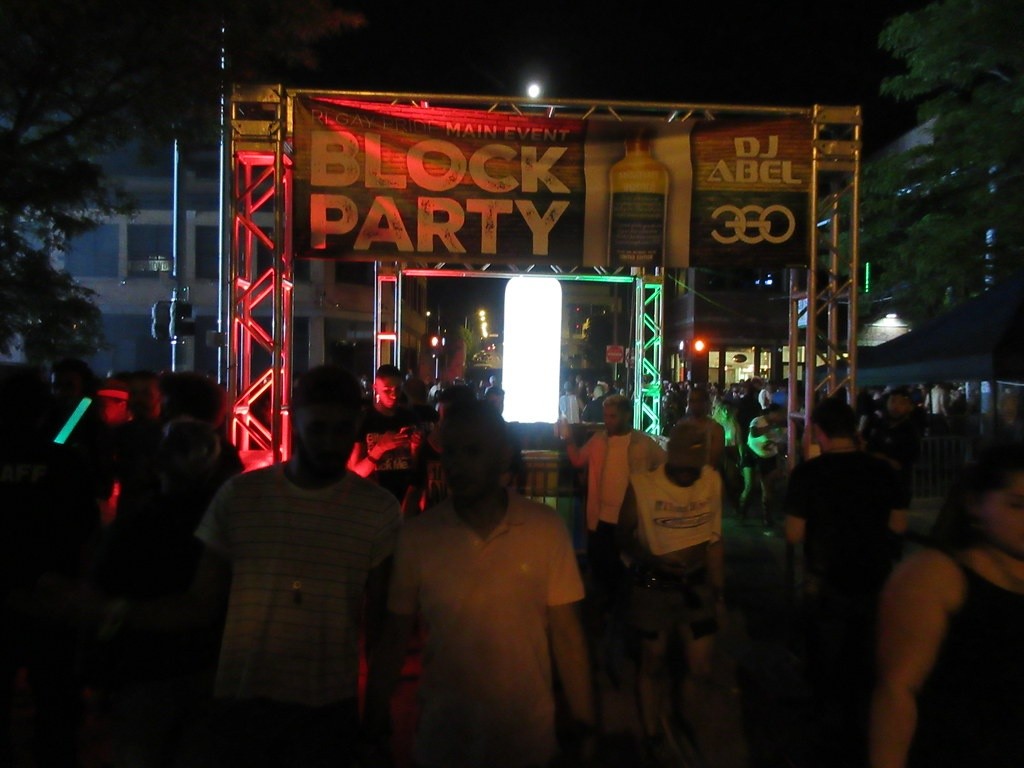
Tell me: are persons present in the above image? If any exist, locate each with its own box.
[50,344,1024,768]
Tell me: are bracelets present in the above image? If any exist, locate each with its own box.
[574,717,598,742]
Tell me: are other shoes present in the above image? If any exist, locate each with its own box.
[761,523,784,537]
[737,498,747,511]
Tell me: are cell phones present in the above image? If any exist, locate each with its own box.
[401,426,417,435]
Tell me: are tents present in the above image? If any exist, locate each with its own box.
[802,269,1024,424]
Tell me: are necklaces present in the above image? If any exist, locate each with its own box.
[825,443,855,452]
[983,536,1024,565]
[282,484,329,605]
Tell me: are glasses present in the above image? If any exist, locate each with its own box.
[887,389,910,398]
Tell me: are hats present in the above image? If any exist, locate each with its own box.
[563,381,572,390]
[775,378,789,387]
[289,366,373,408]
[489,376,496,384]
[96,379,135,401]
